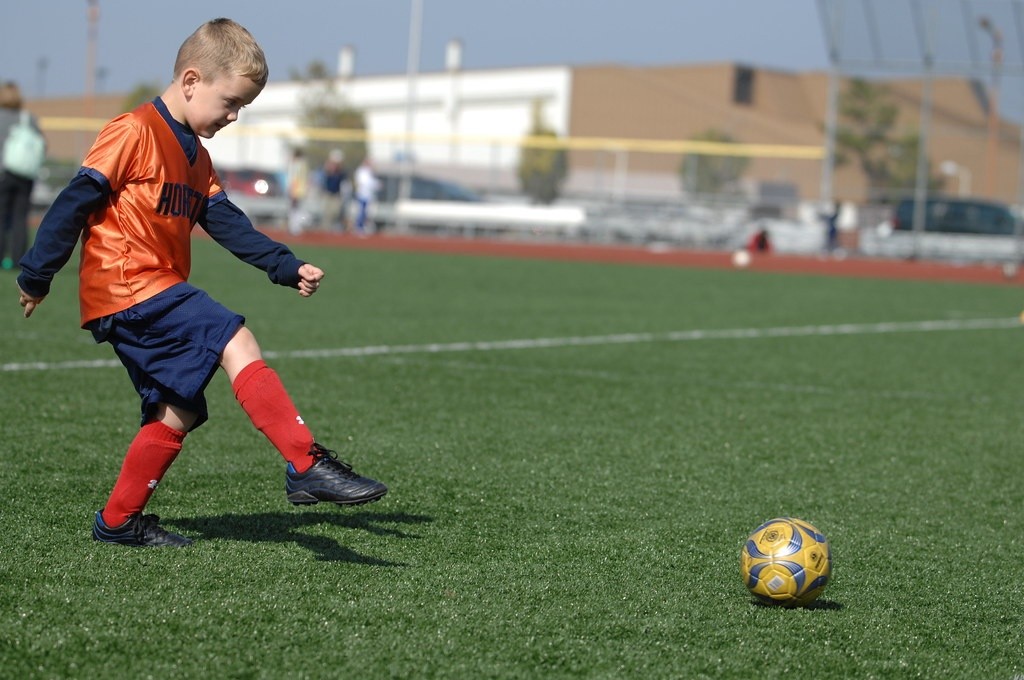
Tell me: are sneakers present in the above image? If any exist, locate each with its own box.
[92,507,194,549]
[284,459,389,506]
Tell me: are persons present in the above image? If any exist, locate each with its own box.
[16,18,389,550]
[0,79,47,272]
[287,147,379,234]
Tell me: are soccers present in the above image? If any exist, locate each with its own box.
[739,518,834,604]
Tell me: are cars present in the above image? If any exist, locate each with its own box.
[292,173,503,236]
[216,168,283,221]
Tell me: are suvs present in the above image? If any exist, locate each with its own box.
[863,190,1020,236]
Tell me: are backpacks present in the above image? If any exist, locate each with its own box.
[1,110,49,181]
